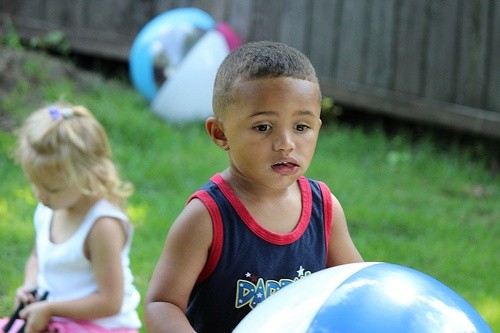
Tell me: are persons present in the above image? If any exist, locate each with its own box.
[0,102,142,333]
[142,39,366,332]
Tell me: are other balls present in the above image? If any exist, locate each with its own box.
[130,6,249,124]
[231,260,495,333]
